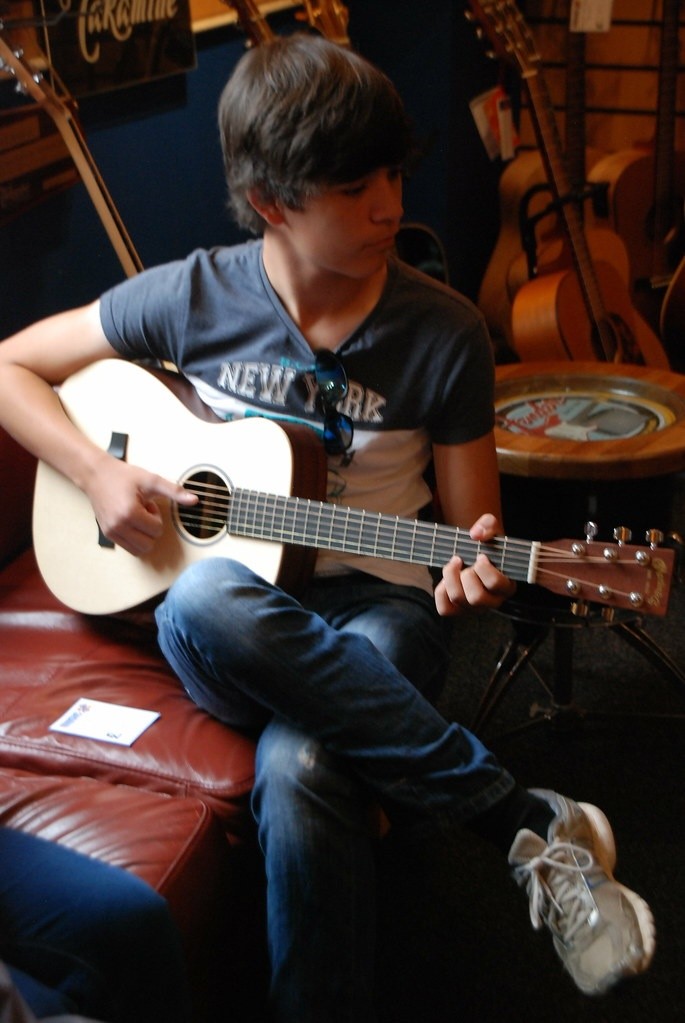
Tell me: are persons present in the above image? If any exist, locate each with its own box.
[0,34,656,1023]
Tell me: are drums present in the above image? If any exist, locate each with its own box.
[484,362,685,623]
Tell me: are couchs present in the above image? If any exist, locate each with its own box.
[1,425,259,943]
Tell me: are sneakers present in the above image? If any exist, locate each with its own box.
[508,788,657,1000]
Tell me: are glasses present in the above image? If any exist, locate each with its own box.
[314,349,354,457]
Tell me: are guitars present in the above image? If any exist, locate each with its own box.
[31,356,685,635]
[0,40,147,280]
[224,0,454,288]
[466,0,685,365]
[293,0,356,40]
